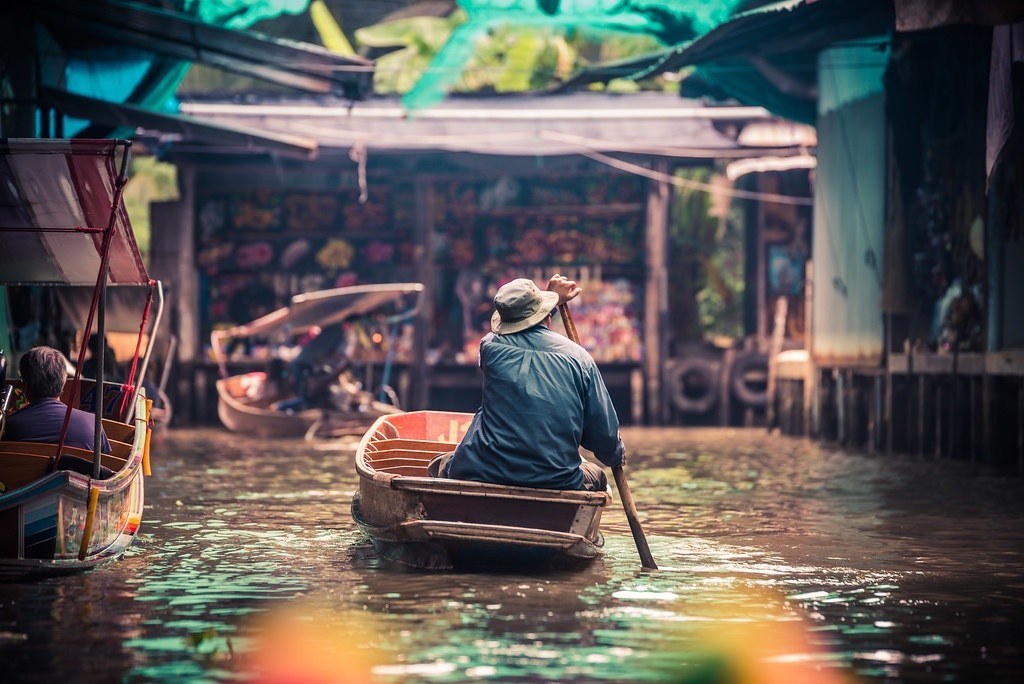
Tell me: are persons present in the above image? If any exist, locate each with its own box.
[426,273,626,492]
[82,332,156,414]
[0,345,112,493]
[268,313,359,413]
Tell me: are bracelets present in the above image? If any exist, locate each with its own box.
[551,307,558,316]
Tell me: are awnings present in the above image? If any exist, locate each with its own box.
[630,0,860,86]
[88,0,374,100]
[535,47,675,97]
[35,83,318,160]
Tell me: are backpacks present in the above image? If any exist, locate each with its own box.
[938,282,986,354]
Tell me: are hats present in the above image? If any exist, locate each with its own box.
[490,278,559,336]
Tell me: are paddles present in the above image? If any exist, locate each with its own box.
[558,300,660,570]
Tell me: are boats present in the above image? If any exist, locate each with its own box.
[211,283,424,438]
[350,410,615,577]
[43,281,179,446]
[0,131,167,575]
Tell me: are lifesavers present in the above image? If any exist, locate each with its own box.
[670,356,720,415]
[732,350,781,407]
